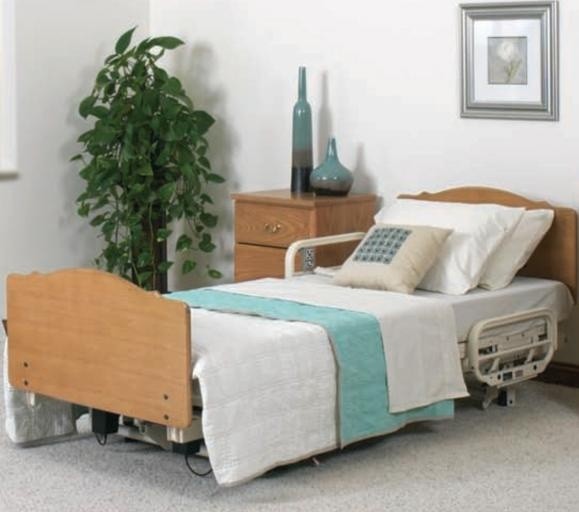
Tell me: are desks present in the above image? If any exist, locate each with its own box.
[229,188,376,283]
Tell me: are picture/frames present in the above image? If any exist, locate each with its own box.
[457,0,560,123]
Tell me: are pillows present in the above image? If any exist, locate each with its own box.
[329,222,454,294]
[476,205,554,291]
[374,198,525,295]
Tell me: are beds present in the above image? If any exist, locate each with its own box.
[5,184,578,487]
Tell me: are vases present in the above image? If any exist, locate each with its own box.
[289,65,311,192]
[309,137,354,197]
